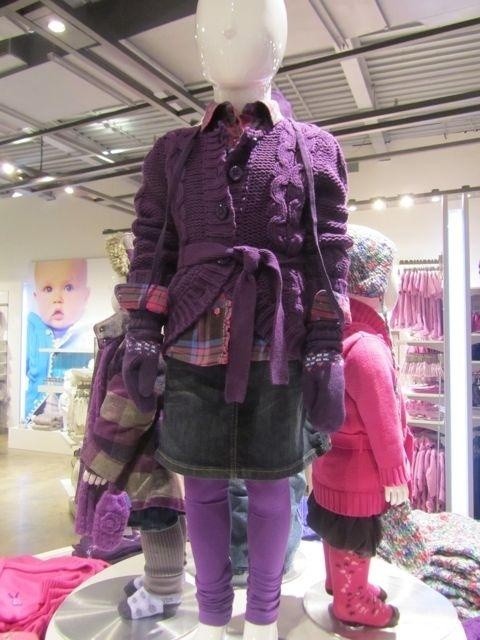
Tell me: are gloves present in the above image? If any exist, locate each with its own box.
[303,341,346,433]
[380,500,429,574]
[91,489,131,552]
[122,309,161,413]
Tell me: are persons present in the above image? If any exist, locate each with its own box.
[115,2,355,640]
[72,231,188,621]
[22,258,98,428]
[305,224,417,630]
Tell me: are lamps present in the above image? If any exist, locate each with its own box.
[347,185,480,211]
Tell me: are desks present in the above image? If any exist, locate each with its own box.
[44,542,467,639]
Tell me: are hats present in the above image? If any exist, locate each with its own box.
[345,223,396,300]
